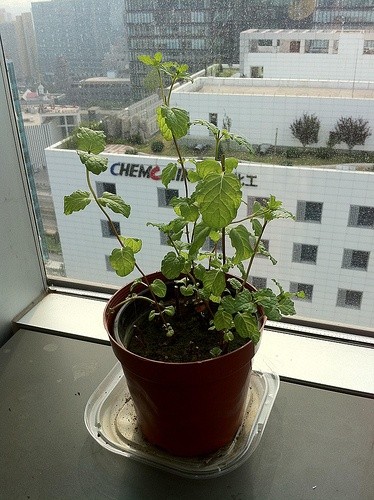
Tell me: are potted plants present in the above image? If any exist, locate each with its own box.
[62,51,310,463]
